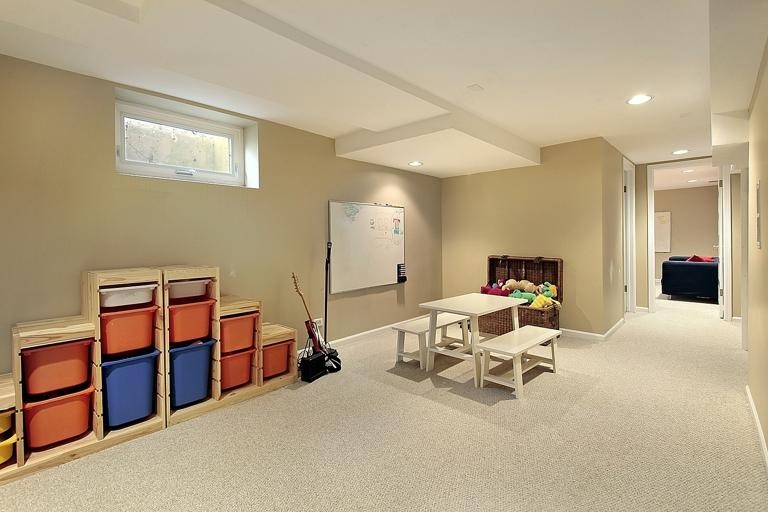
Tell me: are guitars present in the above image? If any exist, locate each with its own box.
[291,272,326,354]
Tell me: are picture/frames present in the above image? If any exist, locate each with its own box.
[655,211,672,253]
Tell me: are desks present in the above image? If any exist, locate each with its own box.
[418,292,528,388]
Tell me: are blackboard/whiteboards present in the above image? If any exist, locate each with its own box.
[328,200,406,295]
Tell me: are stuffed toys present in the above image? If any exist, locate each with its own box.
[480,276,558,309]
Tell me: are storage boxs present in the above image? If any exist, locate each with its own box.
[23,385,94,449]
[1,409,16,435]
[219,312,261,354]
[468,255,563,346]
[169,297,217,343]
[21,338,94,395]
[263,341,292,379]
[101,304,156,355]
[221,348,257,390]
[169,280,210,299]
[170,340,217,408]
[0,435,16,462]
[102,350,160,428]
[98,283,157,308]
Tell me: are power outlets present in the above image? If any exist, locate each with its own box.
[314,318,323,326]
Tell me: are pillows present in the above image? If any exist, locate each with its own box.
[669,255,718,262]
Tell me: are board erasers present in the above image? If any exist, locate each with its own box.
[397,262,407,284]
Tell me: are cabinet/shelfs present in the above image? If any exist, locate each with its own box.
[0,266,166,486]
[157,264,298,428]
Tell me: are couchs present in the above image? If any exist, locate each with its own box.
[662,256,718,300]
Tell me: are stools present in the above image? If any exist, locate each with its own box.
[390,310,479,370]
[476,325,562,398]
